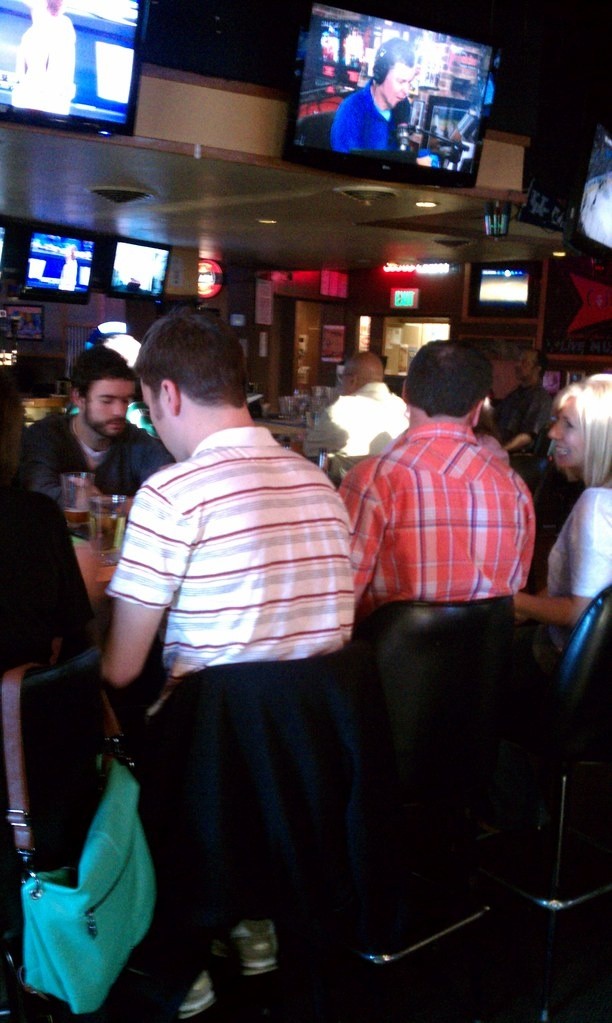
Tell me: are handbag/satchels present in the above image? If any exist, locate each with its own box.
[0,663,159,1014]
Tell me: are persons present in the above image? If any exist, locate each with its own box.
[0,369,95,669]
[484,349,550,456]
[20,347,175,515]
[339,343,536,616]
[303,354,411,487]
[320,27,432,166]
[95,309,359,1017]
[515,372,612,632]
[11,0,77,114]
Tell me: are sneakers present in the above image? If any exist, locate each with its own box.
[212,918,277,976]
[126,954,215,1019]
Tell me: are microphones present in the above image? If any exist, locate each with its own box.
[397,122,412,145]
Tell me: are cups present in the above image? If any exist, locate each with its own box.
[278,386,335,426]
[88,495,127,567]
[62,473,97,526]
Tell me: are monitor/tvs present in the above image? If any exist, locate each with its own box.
[0,0,151,135]
[468,261,540,318]
[0,225,8,271]
[18,225,98,305]
[281,0,500,188]
[104,237,174,303]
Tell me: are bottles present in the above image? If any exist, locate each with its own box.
[318,446,329,475]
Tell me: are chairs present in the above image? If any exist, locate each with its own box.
[461,585,612,909]
[351,596,515,963]
[0,650,134,1021]
[137,636,366,1020]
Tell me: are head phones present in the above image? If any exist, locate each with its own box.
[372,37,411,84]
